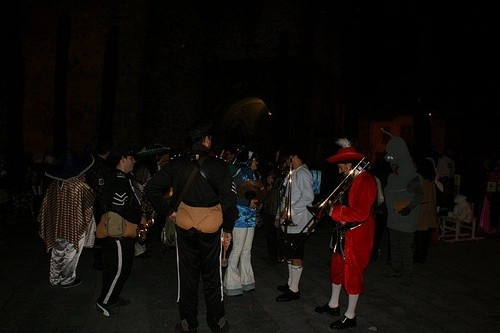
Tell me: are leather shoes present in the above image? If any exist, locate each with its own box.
[314,300,340,316]
[330,314,357,329]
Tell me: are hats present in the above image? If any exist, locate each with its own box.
[236,146,254,168]
[326,138,366,163]
[117,142,137,156]
[45,147,95,180]
[185,121,212,140]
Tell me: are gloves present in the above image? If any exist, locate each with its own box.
[398,207,412,216]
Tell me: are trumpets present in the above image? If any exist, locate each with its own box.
[136,216,159,245]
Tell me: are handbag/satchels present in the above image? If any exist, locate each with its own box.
[161,216,176,247]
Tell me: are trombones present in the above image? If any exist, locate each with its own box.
[289,151,376,252]
[275,158,289,265]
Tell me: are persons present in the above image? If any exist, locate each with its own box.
[380,136,426,290]
[314,146,378,329]
[433,151,500,236]
[37,134,314,316]
[143,123,235,333]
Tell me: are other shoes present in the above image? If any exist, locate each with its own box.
[224,289,242,296]
[109,296,130,307]
[241,282,256,290]
[274,283,290,292]
[94,301,120,319]
[275,289,301,303]
[384,271,415,287]
[61,278,83,288]
